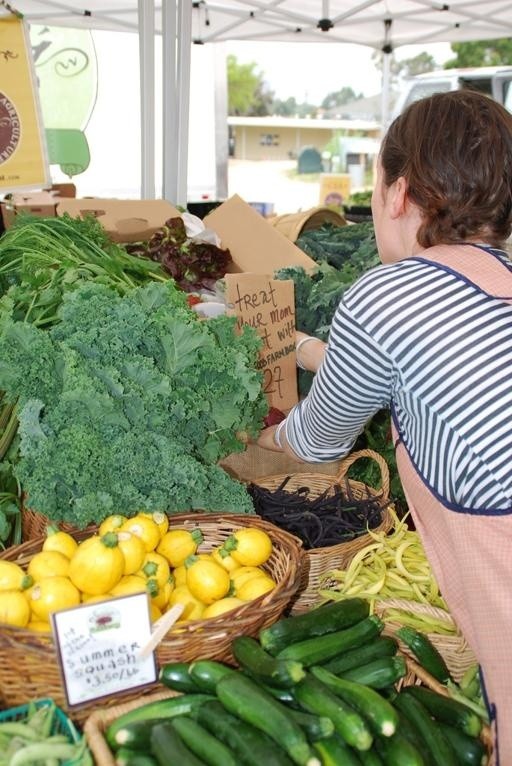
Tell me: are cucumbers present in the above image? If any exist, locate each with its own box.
[108,596,489,766]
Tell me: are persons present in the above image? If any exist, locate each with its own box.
[250,87,511,765]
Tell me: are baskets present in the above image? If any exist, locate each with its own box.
[1,448,495,764]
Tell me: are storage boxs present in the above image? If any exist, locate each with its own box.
[198,193,321,284]
[0,193,68,234]
[54,196,186,244]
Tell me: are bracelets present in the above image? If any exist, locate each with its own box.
[274,418,286,449]
[296,337,321,370]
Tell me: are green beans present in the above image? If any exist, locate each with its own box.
[0,702,92,766]
[323,508,458,634]
